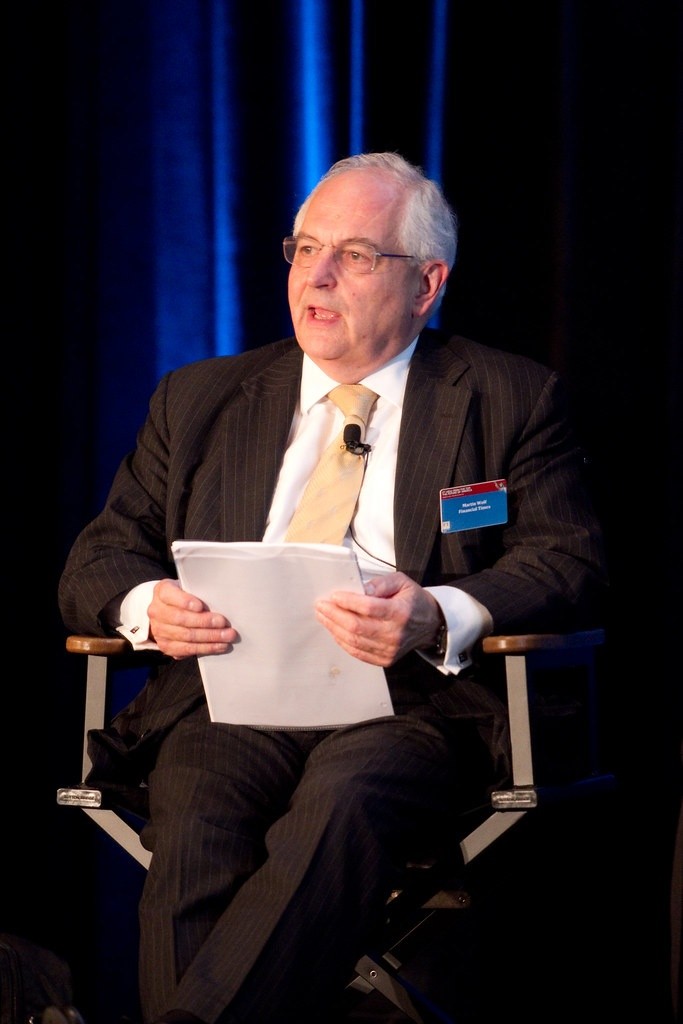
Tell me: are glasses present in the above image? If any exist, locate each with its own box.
[282,236,413,274]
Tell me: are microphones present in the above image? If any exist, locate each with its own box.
[344,424,362,450]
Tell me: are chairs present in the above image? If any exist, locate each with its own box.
[57,630,615,1024]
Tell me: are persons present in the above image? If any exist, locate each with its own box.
[58,153,610,1024]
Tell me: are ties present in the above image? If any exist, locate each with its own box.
[283,384,380,545]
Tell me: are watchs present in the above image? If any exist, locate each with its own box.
[426,597,448,659]
